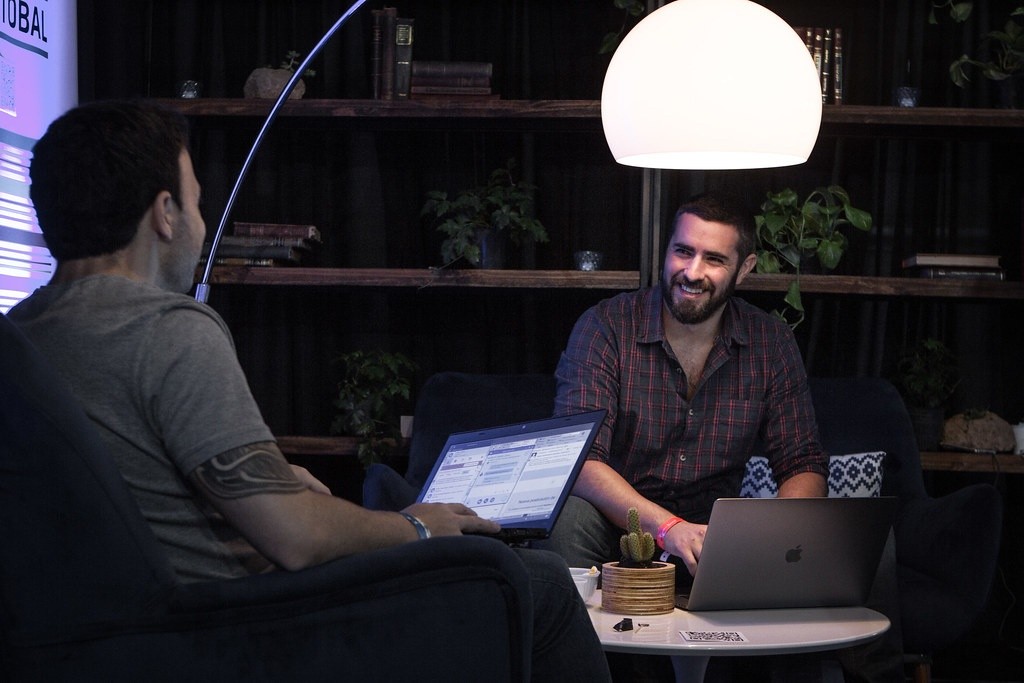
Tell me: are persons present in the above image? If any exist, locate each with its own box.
[5,100,613,683]
[528,192,830,590]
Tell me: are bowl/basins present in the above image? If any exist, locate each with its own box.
[568,566,600,602]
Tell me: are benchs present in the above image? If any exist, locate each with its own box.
[358,370,1004,683]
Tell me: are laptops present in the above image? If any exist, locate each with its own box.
[675,495,900,610]
[416,409,609,540]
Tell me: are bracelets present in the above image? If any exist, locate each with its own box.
[401,512,431,539]
[657,517,687,548]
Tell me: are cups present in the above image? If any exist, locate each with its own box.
[178,80,198,99]
[1011,424,1024,454]
[574,251,603,271]
[893,86,919,108]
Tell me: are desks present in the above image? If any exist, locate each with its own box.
[583,590,890,683]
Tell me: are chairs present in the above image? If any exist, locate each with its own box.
[0,313,535,683]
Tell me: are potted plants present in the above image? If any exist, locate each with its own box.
[601,507,677,617]
[419,159,559,277]
[749,178,871,335]
[899,337,979,451]
[326,349,420,475]
[929,0,1024,107]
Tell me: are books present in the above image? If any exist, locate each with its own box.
[198,222,321,266]
[371,7,493,100]
[902,253,1008,281]
[793,27,842,104]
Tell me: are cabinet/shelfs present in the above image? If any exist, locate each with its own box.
[80,97,1024,476]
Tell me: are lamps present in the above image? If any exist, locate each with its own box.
[193,0,823,302]
